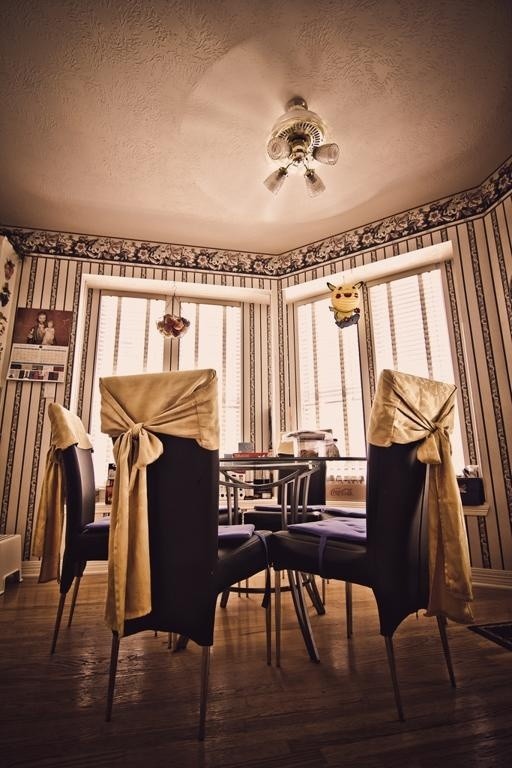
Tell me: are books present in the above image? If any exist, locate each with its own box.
[232,452,268,457]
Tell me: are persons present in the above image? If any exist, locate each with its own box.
[26,311,58,345]
[40,319,56,346]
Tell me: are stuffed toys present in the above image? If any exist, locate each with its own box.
[326,282,363,328]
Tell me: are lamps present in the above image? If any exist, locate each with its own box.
[263,99,342,199]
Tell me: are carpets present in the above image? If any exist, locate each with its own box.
[466,614,511,656]
